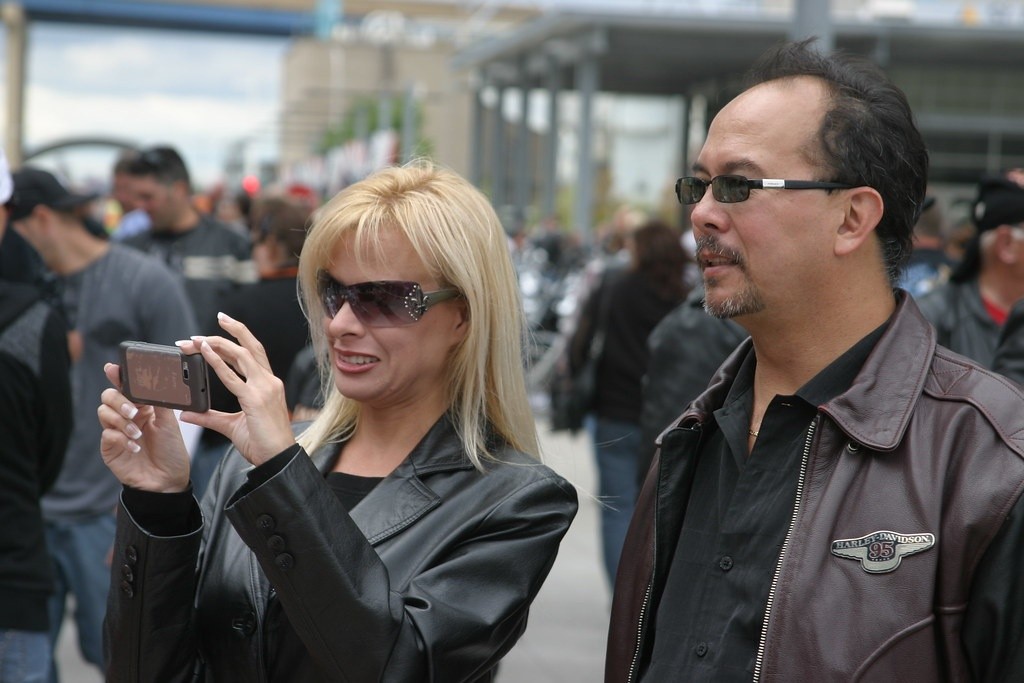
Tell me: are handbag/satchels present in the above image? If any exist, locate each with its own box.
[554,366,593,432]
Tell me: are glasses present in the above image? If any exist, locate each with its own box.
[316,268,464,326]
[675,174,854,205]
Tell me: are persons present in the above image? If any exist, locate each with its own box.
[910,174,1024,387]
[603,38,1024,682]
[114,146,254,499]
[202,184,327,423]
[98,162,577,683]
[0,159,77,683]
[512,208,749,587]
[8,165,205,683]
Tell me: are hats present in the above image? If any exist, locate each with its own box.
[6,169,96,209]
[972,181,1024,237]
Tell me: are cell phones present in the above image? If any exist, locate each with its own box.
[119,341,211,413]
